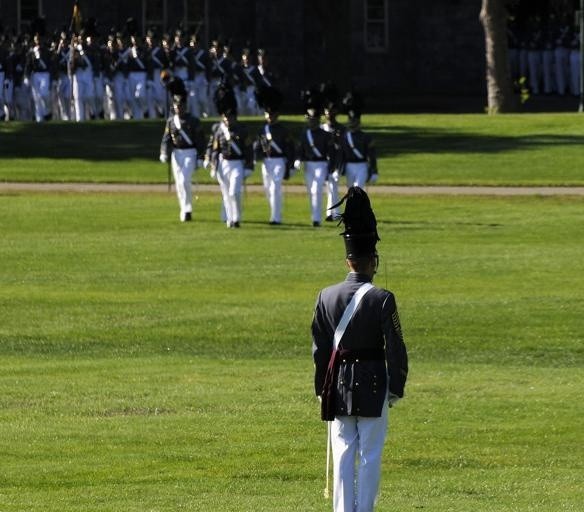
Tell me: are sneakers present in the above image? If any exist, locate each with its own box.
[175,207,337,228]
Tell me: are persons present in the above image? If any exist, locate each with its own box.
[252,106,294,225]
[310,252,408,512]
[332,110,378,189]
[320,102,346,221]
[203,110,227,223]
[210,110,252,228]
[508,10,580,97]
[0,17,279,122]
[159,95,206,221]
[293,108,335,227]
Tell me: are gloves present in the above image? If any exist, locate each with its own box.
[294,159,302,171]
[242,168,253,178]
[369,173,378,183]
[316,395,322,405]
[202,158,211,170]
[159,153,170,165]
[210,169,217,178]
[387,391,402,408]
[332,169,340,182]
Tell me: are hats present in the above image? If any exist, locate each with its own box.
[344,230,379,260]
[170,89,363,120]
